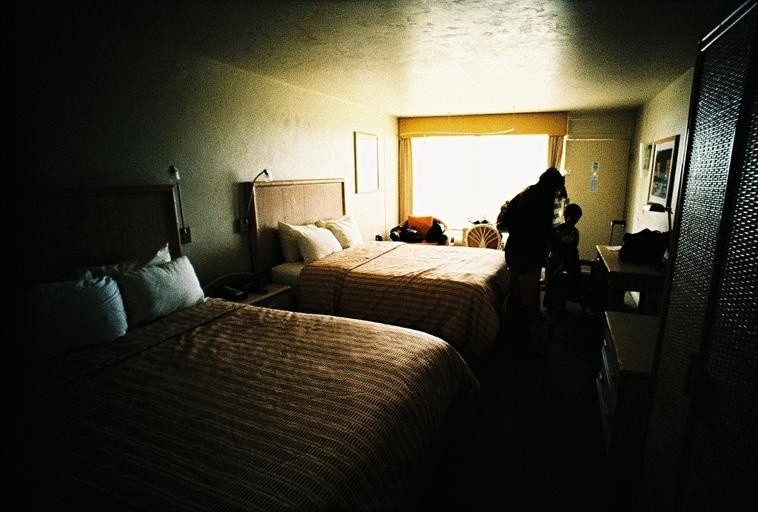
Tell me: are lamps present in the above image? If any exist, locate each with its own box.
[236,167,273,234]
[166,163,192,243]
[646,203,673,230]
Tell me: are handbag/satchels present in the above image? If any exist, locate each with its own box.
[618,228,658,265]
[497,201,510,233]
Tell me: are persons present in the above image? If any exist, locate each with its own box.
[543,204,582,313]
[497,167,576,365]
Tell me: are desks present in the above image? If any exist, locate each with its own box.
[594,244,670,314]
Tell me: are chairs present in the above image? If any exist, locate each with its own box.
[465,224,504,250]
[594,336,664,444]
[390,217,451,245]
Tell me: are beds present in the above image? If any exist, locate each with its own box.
[0,182,499,512]
[237,176,515,371]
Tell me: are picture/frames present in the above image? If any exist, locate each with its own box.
[646,132,680,211]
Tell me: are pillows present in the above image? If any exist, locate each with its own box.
[8,243,207,357]
[266,212,378,266]
[407,215,433,238]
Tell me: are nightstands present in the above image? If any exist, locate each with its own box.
[237,281,294,308]
